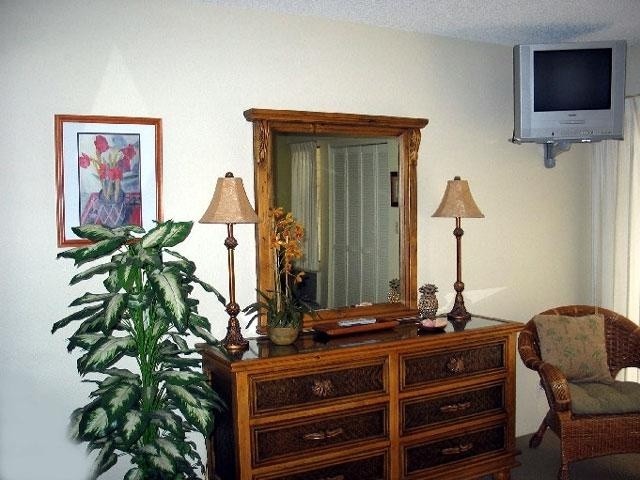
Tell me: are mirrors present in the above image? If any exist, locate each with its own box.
[245,109,429,336]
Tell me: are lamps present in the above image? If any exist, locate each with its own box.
[430,176,485,324]
[198,171,263,351]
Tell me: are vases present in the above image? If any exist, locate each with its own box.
[266,327,300,346]
[101,179,122,205]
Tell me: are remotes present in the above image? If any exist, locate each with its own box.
[338,317,377,327]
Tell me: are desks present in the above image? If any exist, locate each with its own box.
[81,193,140,226]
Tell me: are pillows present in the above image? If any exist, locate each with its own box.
[533,313,614,385]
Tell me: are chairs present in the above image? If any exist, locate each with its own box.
[519,304,640,480]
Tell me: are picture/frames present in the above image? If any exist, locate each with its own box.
[54,114,162,249]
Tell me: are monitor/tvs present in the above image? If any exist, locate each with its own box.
[514,39,627,139]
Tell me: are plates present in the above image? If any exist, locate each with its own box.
[418,318,449,331]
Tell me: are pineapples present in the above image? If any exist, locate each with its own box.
[419,284,438,318]
[387,279,400,303]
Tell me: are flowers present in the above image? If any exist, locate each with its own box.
[277,212,304,297]
[79,134,136,180]
[241,206,304,328]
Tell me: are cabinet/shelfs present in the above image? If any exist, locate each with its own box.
[194,314,526,480]
